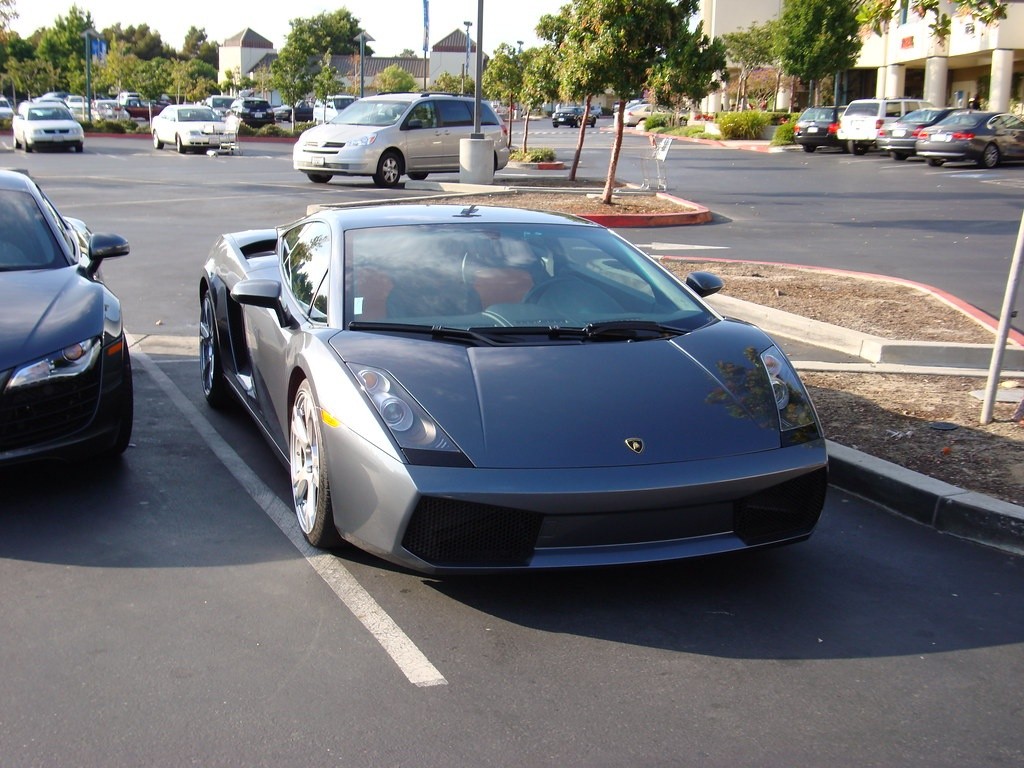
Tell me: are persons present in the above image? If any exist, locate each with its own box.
[225,109,239,134]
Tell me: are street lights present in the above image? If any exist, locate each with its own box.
[460,20,472,96]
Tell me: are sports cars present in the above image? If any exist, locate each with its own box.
[0,168,135,471]
[197,201,832,576]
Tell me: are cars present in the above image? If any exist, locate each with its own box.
[149,105,236,154]
[36,91,90,114]
[312,94,361,127]
[0,96,15,121]
[203,95,236,111]
[113,91,144,105]
[273,98,314,122]
[121,98,166,122]
[916,110,1024,168]
[292,90,512,188]
[161,93,189,107]
[794,104,857,154]
[551,107,597,128]
[613,99,652,116]
[91,99,131,125]
[615,104,688,127]
[10,99,86,154]
[875,105,974,162]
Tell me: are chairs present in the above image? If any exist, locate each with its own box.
[466,240,534,309]
[357,265,397,317]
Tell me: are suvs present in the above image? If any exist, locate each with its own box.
[229,96,276,130]
[838,97,936,157]
